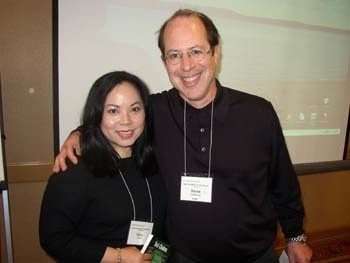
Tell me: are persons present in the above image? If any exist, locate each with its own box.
[52,9,313,263]
[39,71,168,263]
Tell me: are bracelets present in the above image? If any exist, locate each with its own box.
[115,248,121,262]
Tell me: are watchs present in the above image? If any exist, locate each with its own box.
[285,233,308,242]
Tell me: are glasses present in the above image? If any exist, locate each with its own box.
[164,46,213,65]
